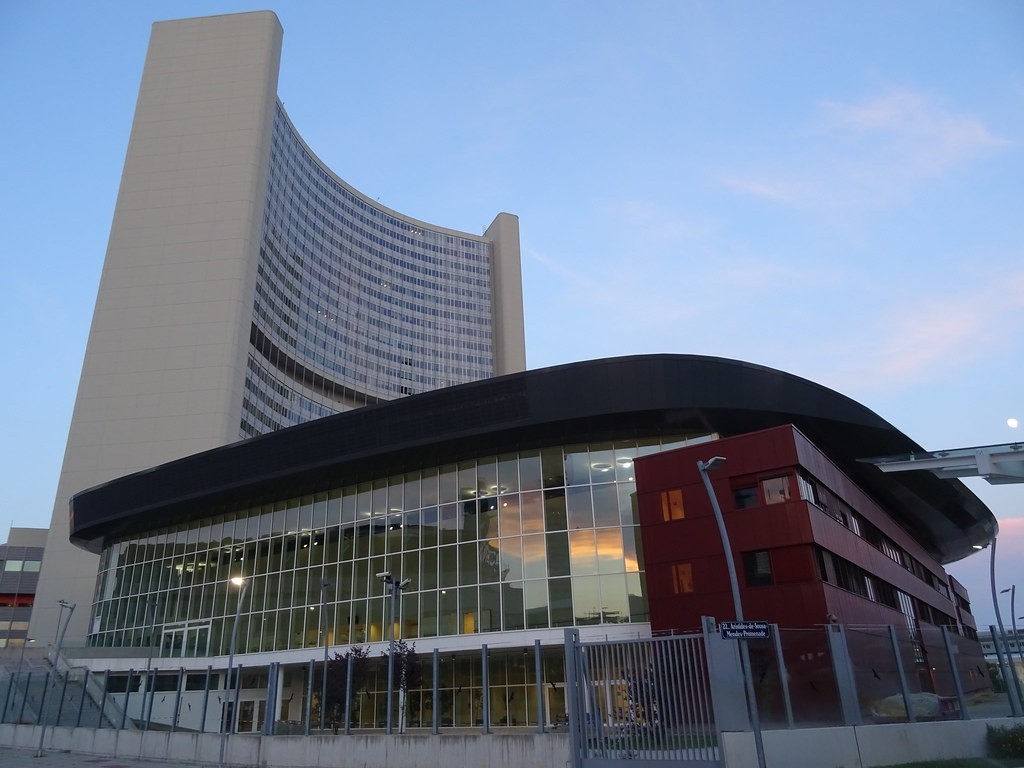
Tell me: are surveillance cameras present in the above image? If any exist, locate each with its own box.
[399,578,411,586]
[376,571,391,577]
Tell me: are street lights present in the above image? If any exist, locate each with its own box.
[697,457,766,768]
[218,577,251,764]
[35,603,77,758]
[972,537,1024,703]
[1000,584,1024,663]
